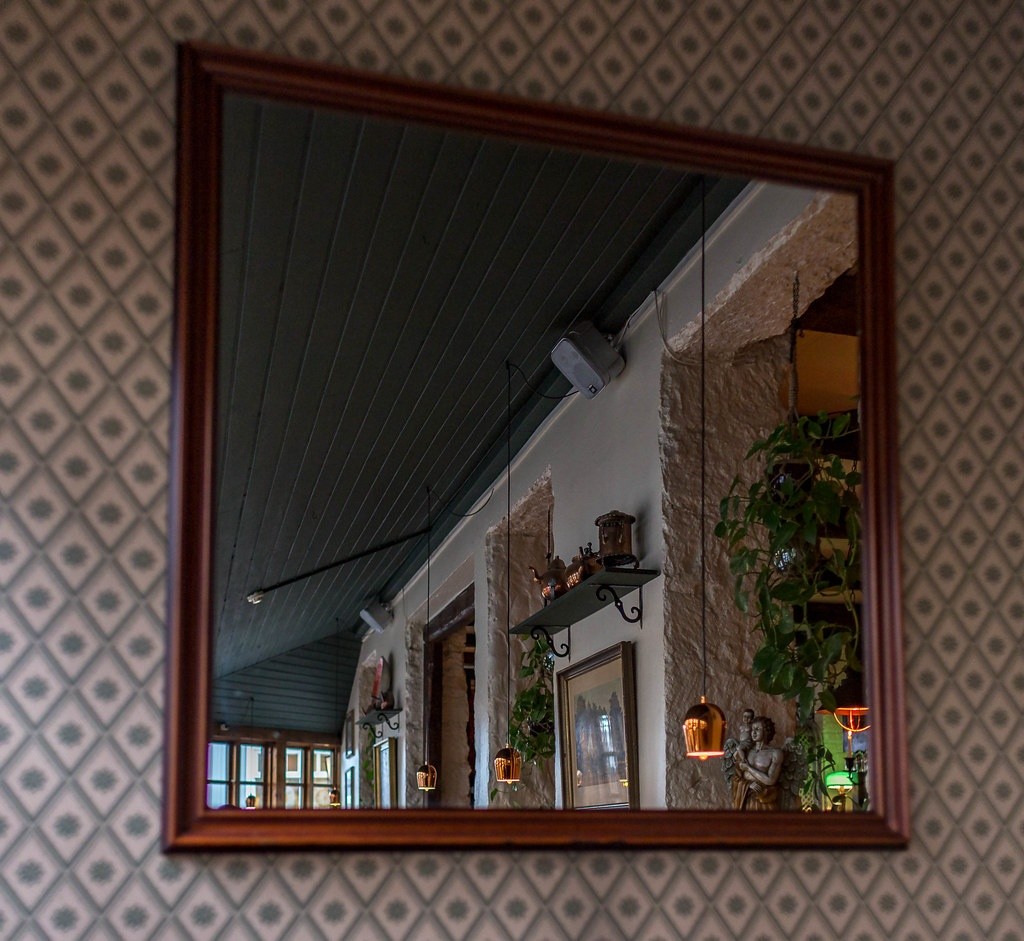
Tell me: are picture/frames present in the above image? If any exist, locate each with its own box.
[344,766,355,810]
[556,641,639,810]
[373,736,398,809]
[344,709,355,757]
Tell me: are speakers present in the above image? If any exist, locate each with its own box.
[551,321,625,400]
[359,596,394,634]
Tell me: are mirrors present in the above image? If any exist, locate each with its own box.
[165,34,913,851]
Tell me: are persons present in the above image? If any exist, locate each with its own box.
[725,709,786,811]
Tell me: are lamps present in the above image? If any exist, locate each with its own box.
[246,696,256,807]
[417,482,437,790]
[330,789,340,807]
[681,173,729,761]
[496,360,521,782]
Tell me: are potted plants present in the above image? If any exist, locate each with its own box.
[717,413,869,811]
[491,630,555,800]
